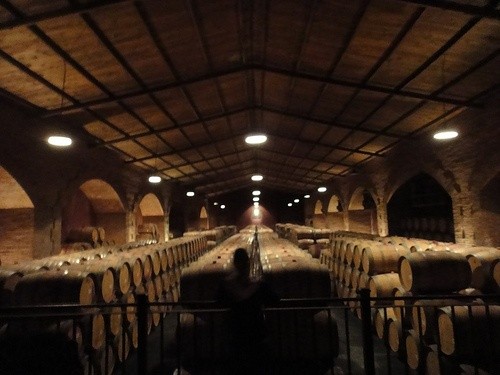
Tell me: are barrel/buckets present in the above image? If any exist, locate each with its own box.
[0,222,500,375]
